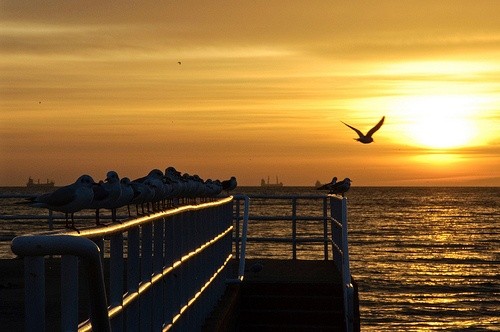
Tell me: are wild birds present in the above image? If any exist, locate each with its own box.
[14,167,237,235]
[317,176,353,197]
[340,116,385,144]
